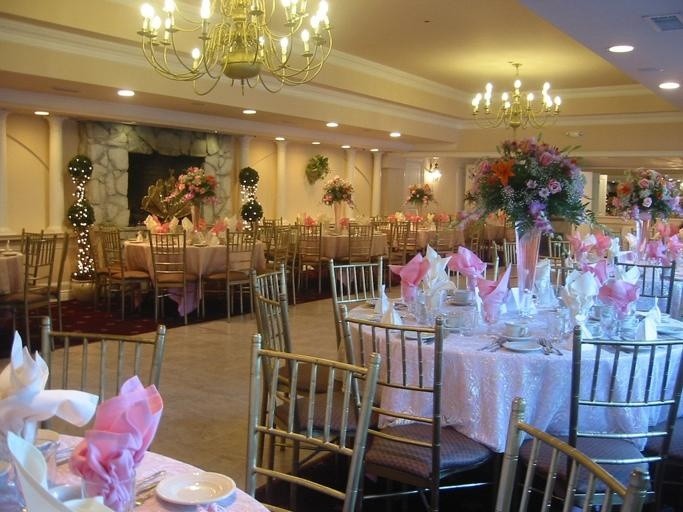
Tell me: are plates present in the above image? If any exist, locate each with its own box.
[367,279,683,355]
[155,472,236,505]
[128,238,256,247]
[35,429,59,446]
[2,251,17,256]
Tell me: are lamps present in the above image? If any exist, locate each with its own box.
[471,60,565,137]
[135,1,339,98]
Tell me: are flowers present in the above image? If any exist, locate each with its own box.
[162,167,222,209]
[450,137,597,242]
[398,182,441,208]
[604,168,683,225]
[319,176,357,210]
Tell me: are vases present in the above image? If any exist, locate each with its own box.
[333,201,345,234]
[632,211,651,266]
[190,205,199,234]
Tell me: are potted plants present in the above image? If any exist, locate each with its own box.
[66,154,100,302]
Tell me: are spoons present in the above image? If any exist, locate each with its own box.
[135,490,156,506]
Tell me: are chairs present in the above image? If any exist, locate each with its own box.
[0,225,70,359]
[26,312,170,451]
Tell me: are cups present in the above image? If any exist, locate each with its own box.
[1,438,135,512]
